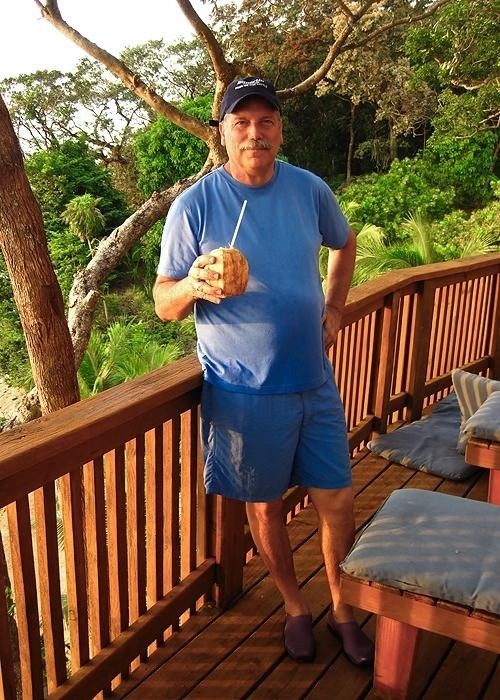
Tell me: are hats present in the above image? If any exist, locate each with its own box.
[218,77,282,121]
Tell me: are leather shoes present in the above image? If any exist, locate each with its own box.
[283,612,318,662]
[324,605,375,666]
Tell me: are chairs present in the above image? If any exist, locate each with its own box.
[465,381,500,506]
[339,485,499,699]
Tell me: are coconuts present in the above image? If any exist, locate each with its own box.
[203,249,248,297]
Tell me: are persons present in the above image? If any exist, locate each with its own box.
[152,76,374,666]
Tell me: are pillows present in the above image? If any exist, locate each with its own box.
[337,489,499,613]
[452,368,500,454]
